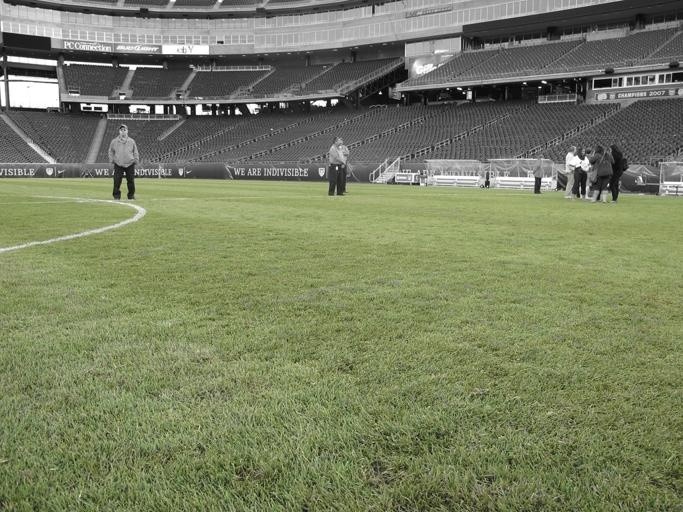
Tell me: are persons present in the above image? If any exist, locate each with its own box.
[338,136,350,193]
[563,144,624,202]
[109,123,140,201]
[532,153,544,193]
[326,136,347,197]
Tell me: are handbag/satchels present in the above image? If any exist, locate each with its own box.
[623,159,628,171]
[588,168,598,184]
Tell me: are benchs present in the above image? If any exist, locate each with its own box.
[0,29,683,190]
